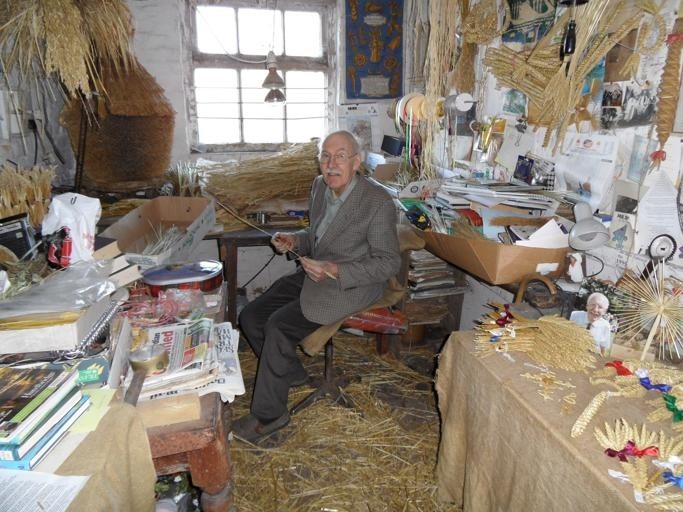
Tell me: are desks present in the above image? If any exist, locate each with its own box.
[203,224,305,329]
[0,280,234,512]
[433,318,683,512]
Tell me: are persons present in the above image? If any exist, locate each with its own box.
[566,252,584,283]
[229,131,402,442]
[570,292,610,358]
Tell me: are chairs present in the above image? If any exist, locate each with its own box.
[289,224,425,416]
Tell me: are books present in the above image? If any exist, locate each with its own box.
[110,253,130,276]
[93,235,122,259]
[252,199,309,228]
[1,293,119,362]
[366,152,403,171]
[0,367,93,472]
[408,250,471,299]
[397,176,581,249]
[108,260,144,290]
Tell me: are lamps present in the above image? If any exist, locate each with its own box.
[568,201,611,278]
[262,50,284,89]
[264,89,287,103]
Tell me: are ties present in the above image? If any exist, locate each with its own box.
[585,323,592,331]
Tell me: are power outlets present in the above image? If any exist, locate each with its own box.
[21,112,35,134]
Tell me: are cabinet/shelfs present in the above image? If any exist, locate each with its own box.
[379,248,465,354]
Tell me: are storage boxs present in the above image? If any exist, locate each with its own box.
[367,162,442,217]
[401,210,569,286]
[98,195,216,270]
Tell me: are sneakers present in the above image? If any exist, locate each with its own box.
[229,414,290,442]
[289,374,312,391]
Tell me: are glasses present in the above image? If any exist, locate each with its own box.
[316,150,358,163]
